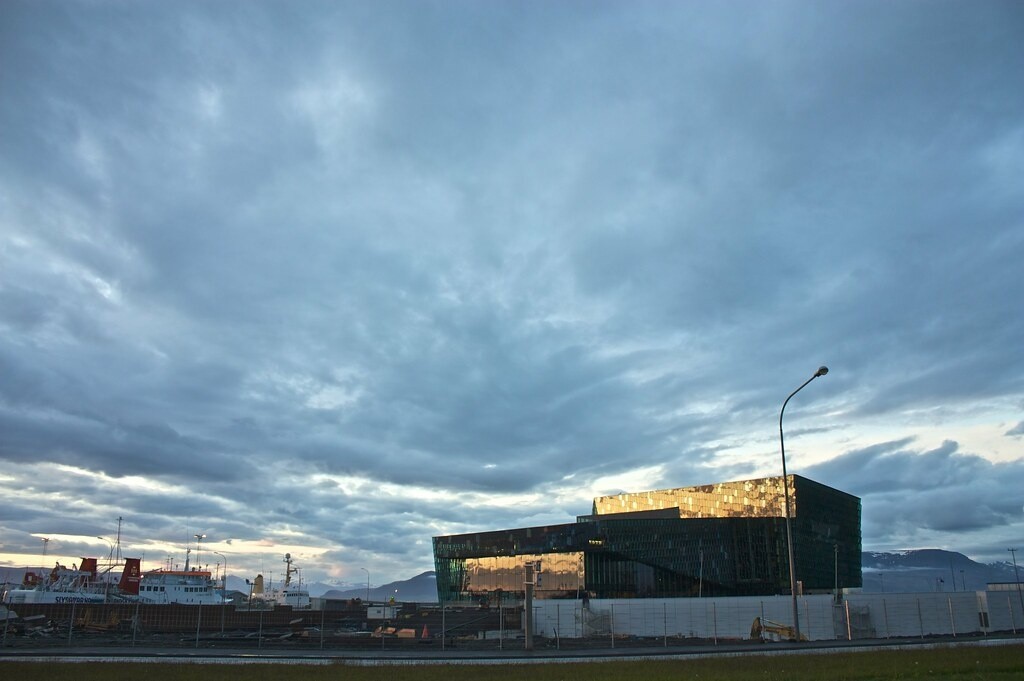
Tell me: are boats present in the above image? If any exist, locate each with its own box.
[3,544,233,620]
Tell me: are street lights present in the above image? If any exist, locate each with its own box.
[361,568,370,607]
[193,535,207,571]
[214,551,227,603]
[38,538,52,578]
[97,536,113,604]
[778,366,830,642]
